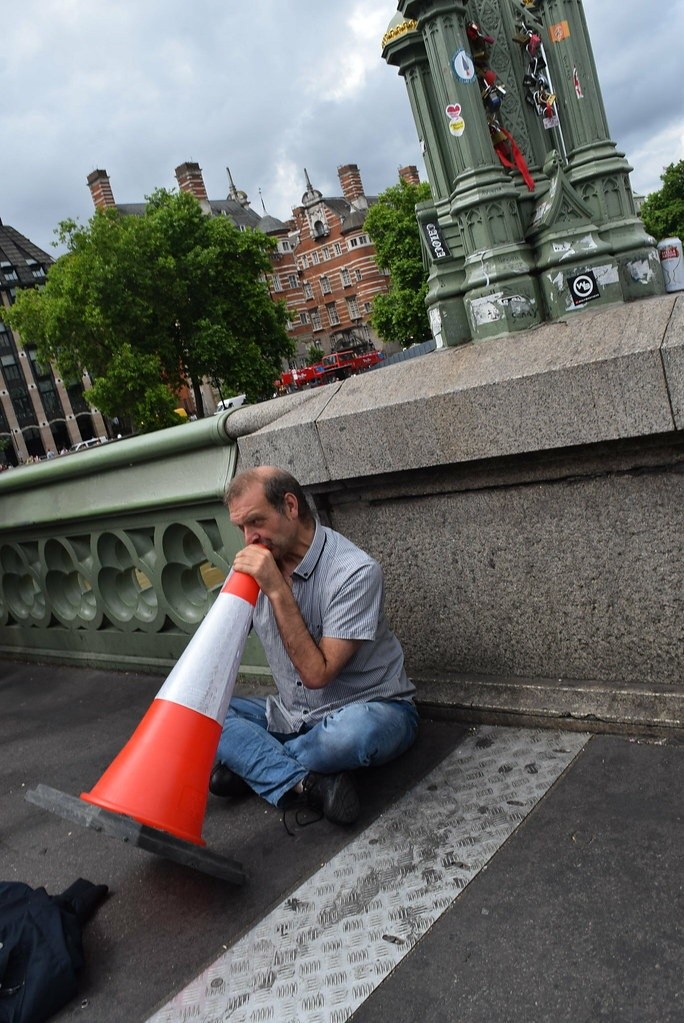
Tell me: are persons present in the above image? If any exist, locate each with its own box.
[205,466,425,836]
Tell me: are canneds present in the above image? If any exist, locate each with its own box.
[657,236,684,293]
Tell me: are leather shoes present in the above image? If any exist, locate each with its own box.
[210,761,248,795]
[283,769,361,836]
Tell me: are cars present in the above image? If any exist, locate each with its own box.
[67,409,198,454]
[213,349,386,417]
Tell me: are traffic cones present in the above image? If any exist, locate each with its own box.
[24,541,269,886]
